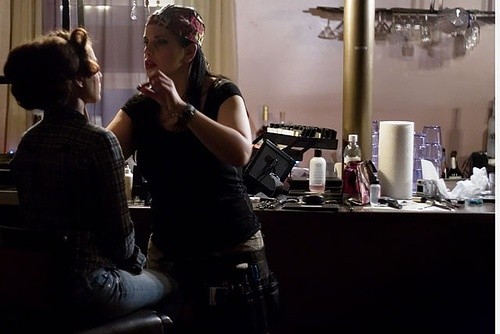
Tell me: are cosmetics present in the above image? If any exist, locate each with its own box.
[123,163,134,206]
[310,149,328,195]
[266,124,337,140]
[370,180,380,205]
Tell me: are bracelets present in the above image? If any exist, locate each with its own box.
[176,99,196,123]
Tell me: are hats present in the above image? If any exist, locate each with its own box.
[145,4,205,47]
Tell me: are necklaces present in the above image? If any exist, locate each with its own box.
[165,83,189,119]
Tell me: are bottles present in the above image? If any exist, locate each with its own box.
[370,175,381,205]
[416,181,424,197]
[124,165,134,206]
[279,111,285,125]
[447,151,463,178]
[343,135,361,195]
[256,107,270,136]
[309,150,326,193]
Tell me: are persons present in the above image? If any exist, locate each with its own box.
[2,27,184,329]
[105,5,282,334]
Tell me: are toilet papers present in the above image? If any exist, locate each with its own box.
[380,120,414,200]
